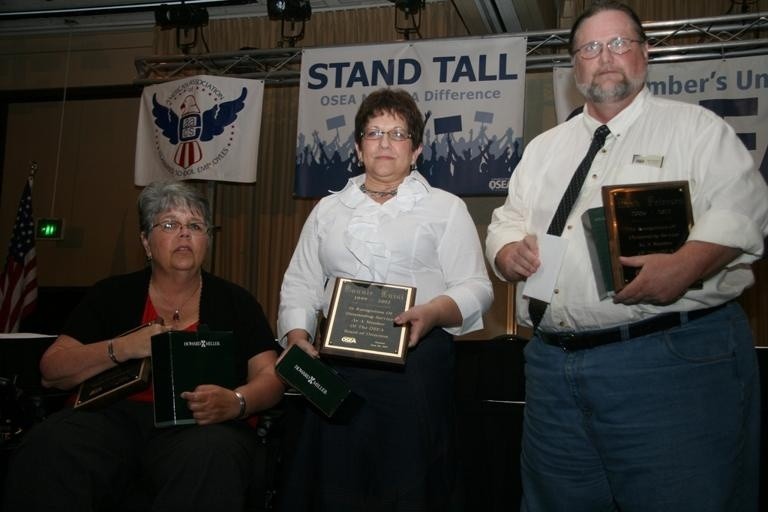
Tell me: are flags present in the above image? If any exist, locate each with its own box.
[0,175,40,335]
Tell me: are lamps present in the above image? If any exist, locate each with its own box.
[153,3,208,47]
[265,1,311,44]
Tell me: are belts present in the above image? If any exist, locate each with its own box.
[539,304,722,352]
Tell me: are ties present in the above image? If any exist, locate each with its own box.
[529,125,611,327]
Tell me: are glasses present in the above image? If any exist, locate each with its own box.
[570,38,641,60]
[361,127,412,141]
[149,221,208,235]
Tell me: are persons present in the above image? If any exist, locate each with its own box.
[4,178,285,512]
[484,2,768,511]
[277,90,495,508]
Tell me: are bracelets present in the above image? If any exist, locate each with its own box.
[107,339,121,365]
[234,391,247,421]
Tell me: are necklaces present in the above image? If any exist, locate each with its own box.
[361,183,398,198]
[150,279,202,321]
